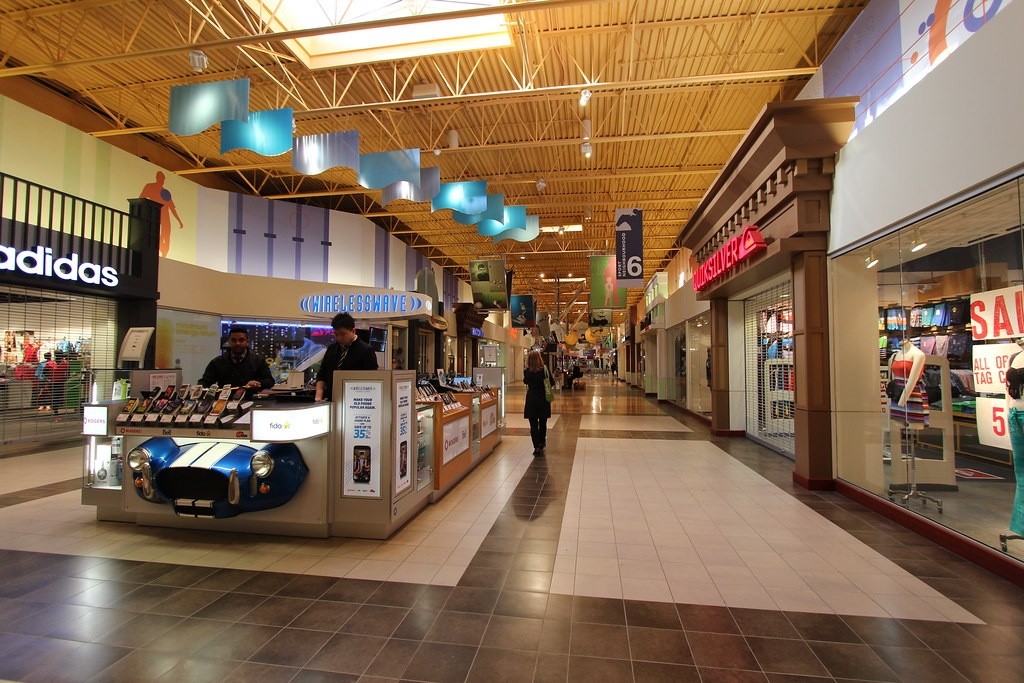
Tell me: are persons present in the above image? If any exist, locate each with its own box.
[197,327,275,400]
[1004,339,1024,536]
[314,314,378,402]
[705,348,712,391]
[886,339,925,429]
[611,362,615,375]
[4,331,82,411]
[567,364,580,390]
[523,350,554,457]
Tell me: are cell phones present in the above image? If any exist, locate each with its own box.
[122,384,245,416]
[400,441,407,478]
[415,382,491,406]
[353,445,371,483]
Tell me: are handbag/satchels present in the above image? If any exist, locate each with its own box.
[543,364,555,402]
[885,380,904,403]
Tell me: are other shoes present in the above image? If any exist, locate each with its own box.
[45,405,51,410]
[37,406,43,410]
[533,448,544,457]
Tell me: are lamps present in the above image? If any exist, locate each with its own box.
[582,119,590,139]
[863,253,879,269]
[906,232,927,252]
[581,142,591,156]
[580,88,591,105]
[189,49,207,72]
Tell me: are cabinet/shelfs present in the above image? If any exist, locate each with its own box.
[915,411,1014,466]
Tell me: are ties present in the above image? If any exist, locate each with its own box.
[336,348,349,370]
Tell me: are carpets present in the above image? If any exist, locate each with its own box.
[954,467,1004,479]
